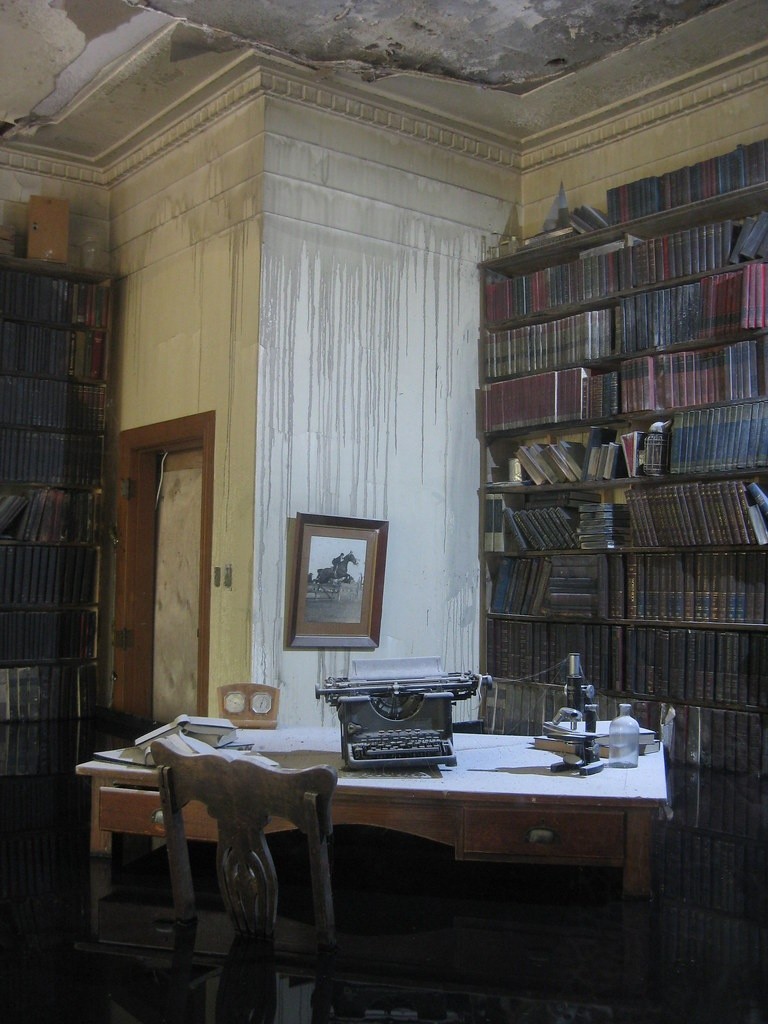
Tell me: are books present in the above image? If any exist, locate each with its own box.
[483,139,765,1022]
[0,269,116,777]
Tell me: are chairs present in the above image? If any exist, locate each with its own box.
[147,737,341,927]
[219,684,280,732]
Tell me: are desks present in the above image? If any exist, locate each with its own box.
[75,733,670,902]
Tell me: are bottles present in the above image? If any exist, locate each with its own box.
[609,704,639,768]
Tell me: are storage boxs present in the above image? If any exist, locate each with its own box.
[24,194,70,266]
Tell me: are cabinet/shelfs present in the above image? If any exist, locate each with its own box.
[0,257,125,899]
[476,180,768,925]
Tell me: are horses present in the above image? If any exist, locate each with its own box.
[318,550,357,591]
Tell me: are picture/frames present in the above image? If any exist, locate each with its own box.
[282,510,390,650]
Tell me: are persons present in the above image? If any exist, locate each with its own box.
[332,553,344,578]
